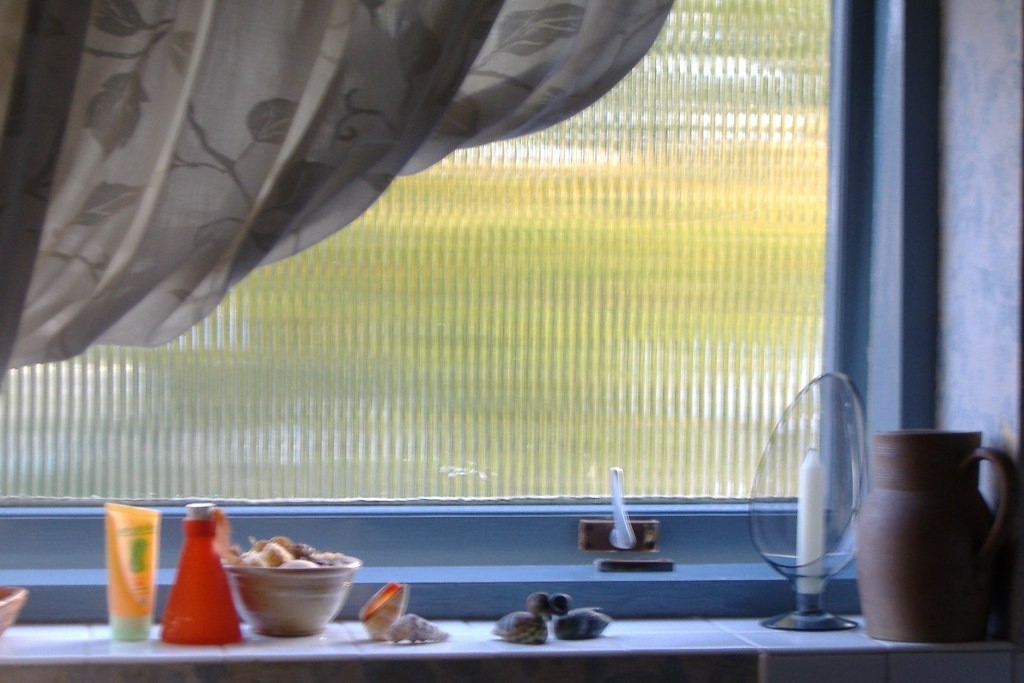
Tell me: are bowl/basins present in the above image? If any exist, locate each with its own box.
[223,552,363,637]
[0,587,30,638]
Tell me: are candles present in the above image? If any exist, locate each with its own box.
[796,448,825,596]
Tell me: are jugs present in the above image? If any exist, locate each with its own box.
[857,432,1019,644]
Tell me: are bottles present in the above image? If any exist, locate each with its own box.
[158,503,245,647]
